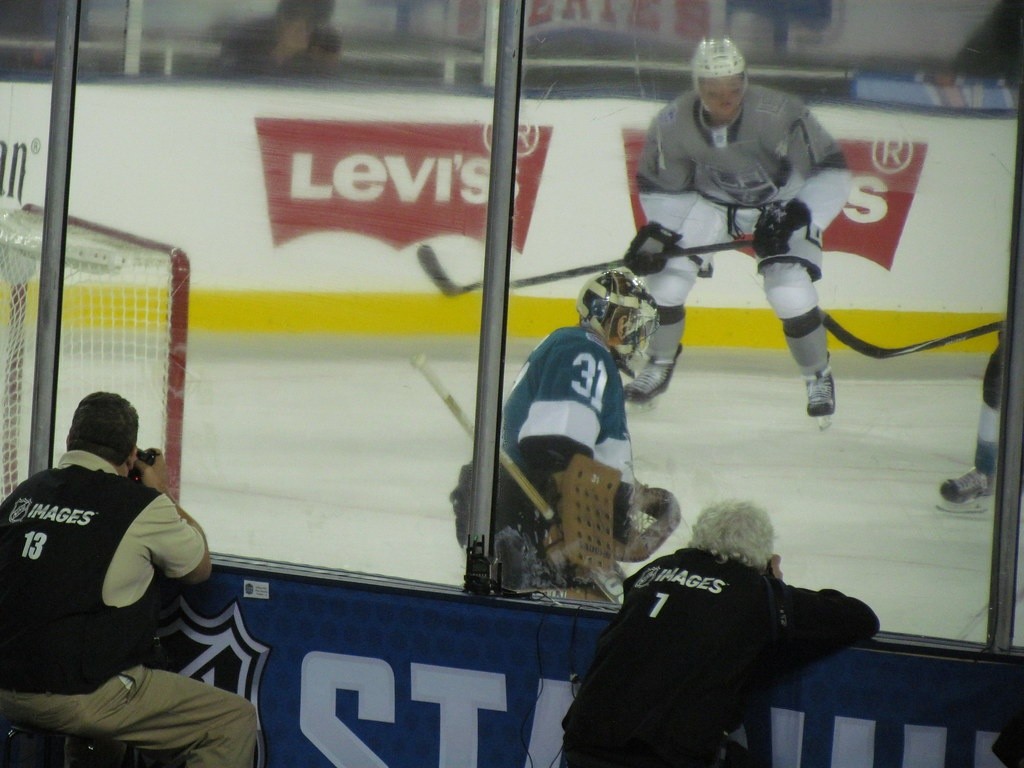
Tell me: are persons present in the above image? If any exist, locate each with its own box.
[938,332,1006,513]
[0,391,257,768]
[934,0,1024,89]
[623,36,852,431]
[224,0,343,80]
[558,501,880,768]
[450,271,680,601]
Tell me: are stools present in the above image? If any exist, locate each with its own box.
[3,723,96,768]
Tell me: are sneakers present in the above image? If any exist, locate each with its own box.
[625,344,683,412]
[804,351,836,429]
[936,468,996,513]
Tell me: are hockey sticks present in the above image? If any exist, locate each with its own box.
[416,238,755,298]
[408,350,628,606]
[824,313,1005,359]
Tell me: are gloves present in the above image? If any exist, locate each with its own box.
[752,198,811,258]
[623,222,683,276]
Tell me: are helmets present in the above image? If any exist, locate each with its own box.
[580,266,664,378]
[691,37,745,91]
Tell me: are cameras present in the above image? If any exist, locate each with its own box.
[128,448,157,484]
